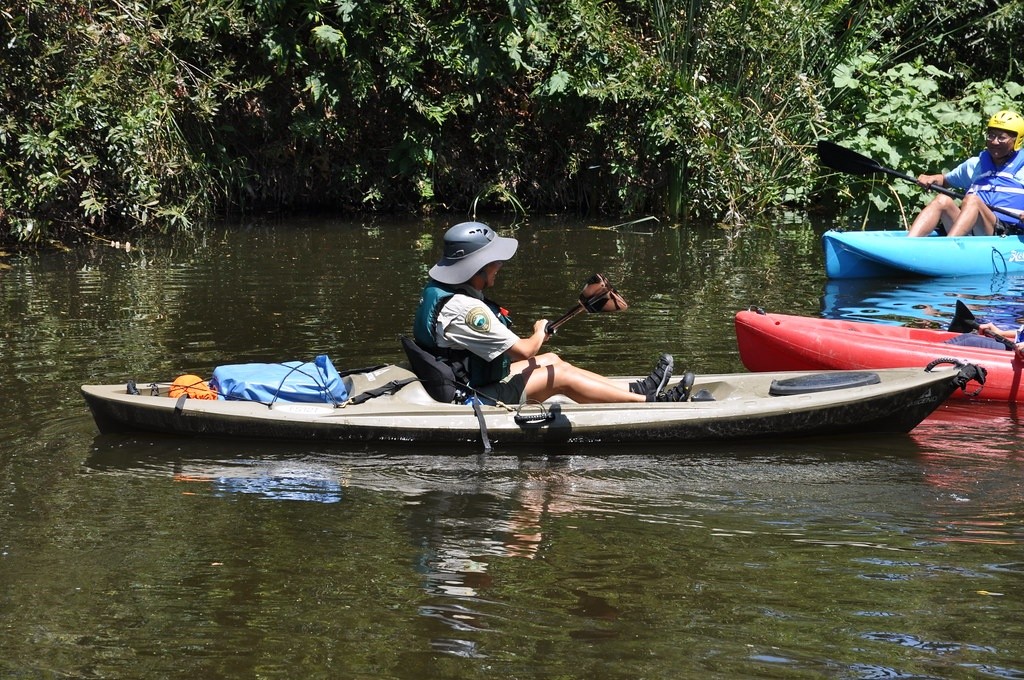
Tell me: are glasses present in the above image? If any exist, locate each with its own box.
[986,132,1016,142]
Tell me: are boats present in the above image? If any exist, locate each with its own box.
[736,306,1024,406]
[818,226,1024,279]
[77,357,995,454]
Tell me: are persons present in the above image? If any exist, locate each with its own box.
[413,223,697,404]
[907,109,1024,237]
[978,320,1024,353]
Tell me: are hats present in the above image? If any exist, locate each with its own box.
[428,222,519,284]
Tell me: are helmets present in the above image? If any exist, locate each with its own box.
[987,110,1024,151]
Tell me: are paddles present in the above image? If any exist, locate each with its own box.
[816,138,1024,224]
[545,272,628,336]
[948,299,1023,358]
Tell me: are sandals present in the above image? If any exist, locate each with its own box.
[638,353,673,396]
[661,373,695,402]
